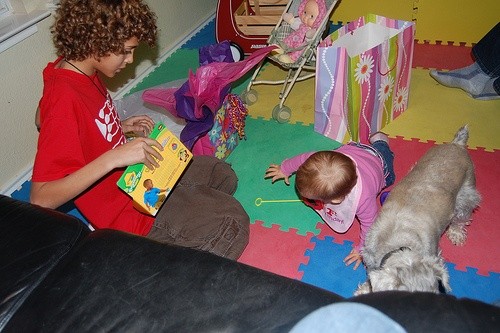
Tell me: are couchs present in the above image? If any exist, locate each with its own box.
[0,194,349,333]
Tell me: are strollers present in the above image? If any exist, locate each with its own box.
[241,0,337,124]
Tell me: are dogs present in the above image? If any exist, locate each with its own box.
[354,125,481,296]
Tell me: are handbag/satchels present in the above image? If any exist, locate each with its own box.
[313,11,417,146]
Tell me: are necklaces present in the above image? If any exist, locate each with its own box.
[64,59,111,103]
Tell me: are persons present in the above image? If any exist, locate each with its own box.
[143,179,167,210]
[429,21,500,99]
[30,0,250,260]
[265,131,395,270]
[272,0,326,64]
